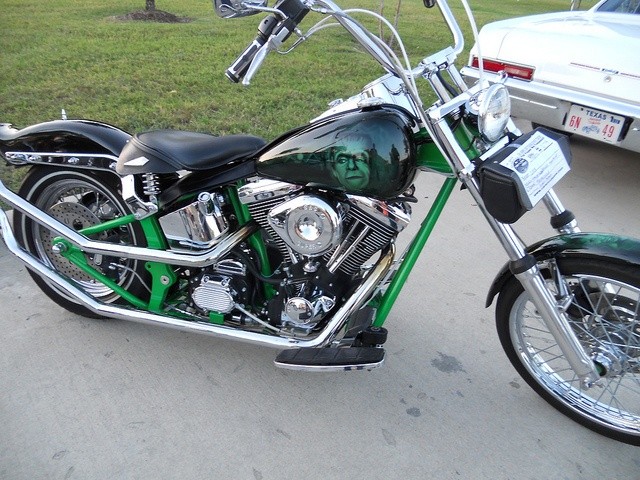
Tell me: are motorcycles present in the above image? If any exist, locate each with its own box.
[0,0,640,447]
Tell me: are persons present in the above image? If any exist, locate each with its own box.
[329,129,373,191]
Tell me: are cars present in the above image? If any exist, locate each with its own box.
[461,0,640,152]
